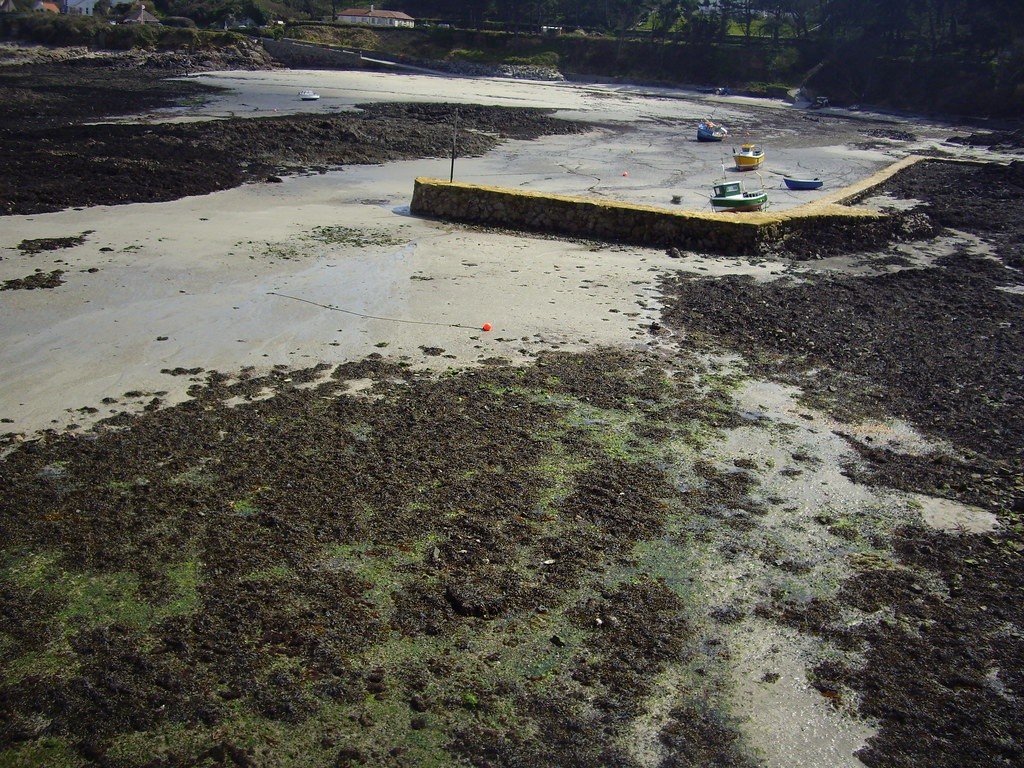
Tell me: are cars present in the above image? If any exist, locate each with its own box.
[812,97,829,108]
[696,82,726,94]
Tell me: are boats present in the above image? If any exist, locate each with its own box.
[732,143,766,171]
[783,177,824,190]
[697,118,728,141]
[298,90,321,99]
[710,180,768,212]
